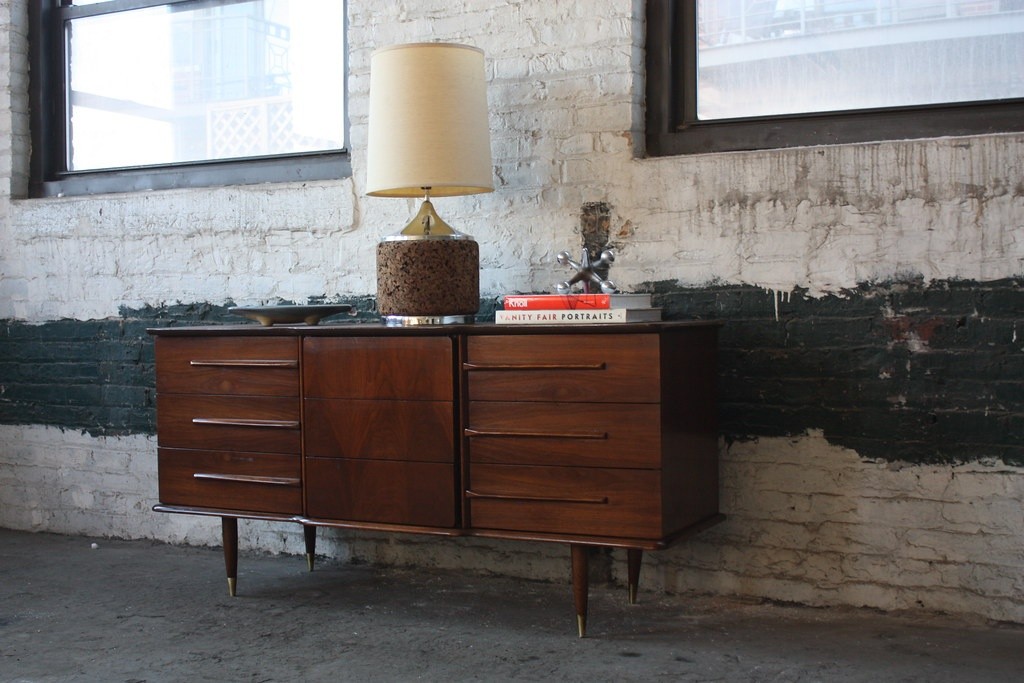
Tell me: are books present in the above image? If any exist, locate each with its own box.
[495,294,663,325]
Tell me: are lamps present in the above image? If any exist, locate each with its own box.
[364,41,496,326]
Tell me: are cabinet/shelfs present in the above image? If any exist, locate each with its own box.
[145,318,725,639]
